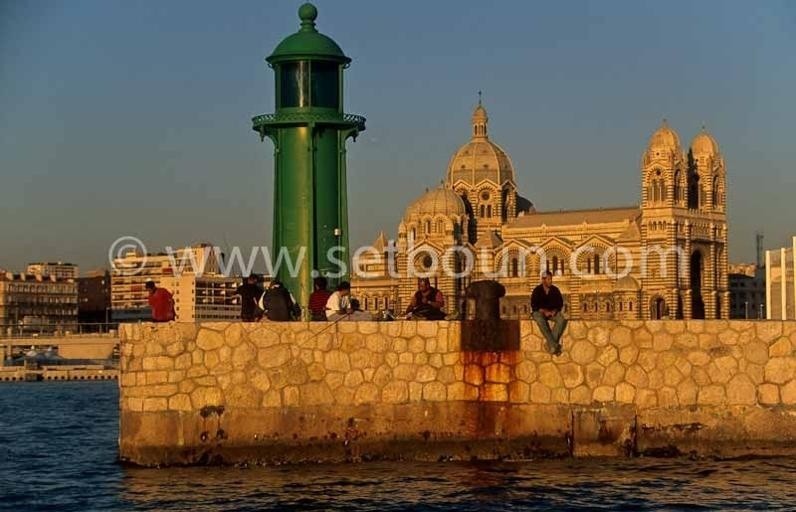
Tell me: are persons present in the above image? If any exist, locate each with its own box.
[306,276,328,320]
[405,277,449,320]
[231,272,262,322]
[257,278,298,322]
[325,281,383,321]
[531,269,566,358]
[144,280,175,321]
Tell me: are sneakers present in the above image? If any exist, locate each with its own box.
[543,342,562,356]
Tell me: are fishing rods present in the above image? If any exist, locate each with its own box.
[298,313,349,348]
